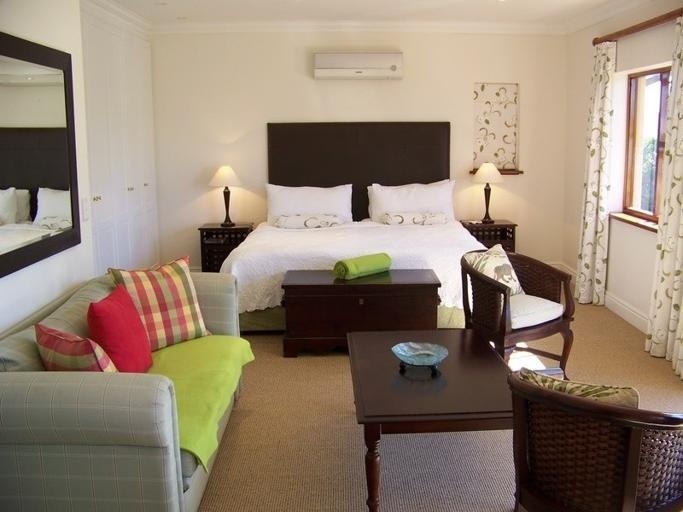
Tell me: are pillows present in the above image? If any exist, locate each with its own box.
[33,324,116,373]
[87,286,154,372]
[472,244,524,297]
[518,367,641,410]
[333,253,391,280]
[0,186,72,230]
[264,178,456,227]
[109,258,206,350]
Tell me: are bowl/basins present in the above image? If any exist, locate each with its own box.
[389,340,448,365]
[391,367,449,396]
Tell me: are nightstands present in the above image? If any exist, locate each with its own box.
[460,218,518,254]
[198,222,255,273]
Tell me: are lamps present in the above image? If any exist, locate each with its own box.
[473,163,503,224]
[210,166,242,226]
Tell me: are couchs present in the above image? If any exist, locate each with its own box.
[0,272,244,512]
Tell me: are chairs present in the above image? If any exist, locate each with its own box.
[461,249,575,381]
[507,368,683,512]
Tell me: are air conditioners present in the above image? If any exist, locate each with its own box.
[313,50,403,81]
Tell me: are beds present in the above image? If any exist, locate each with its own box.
[0,126,68,254]
[222,122,490,333]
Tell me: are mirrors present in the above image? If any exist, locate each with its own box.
[0,32,83,279]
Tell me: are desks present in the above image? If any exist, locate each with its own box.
[282,269,442,358]
